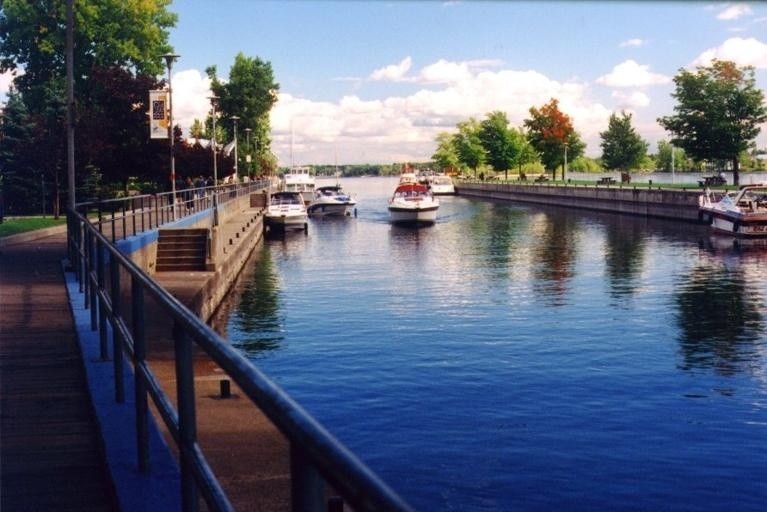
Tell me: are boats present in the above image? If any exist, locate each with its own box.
[695,181,767,238]
[265,185,312,234]
[280,126,319,204]
[308,149,359,218]
[385,161,458,224]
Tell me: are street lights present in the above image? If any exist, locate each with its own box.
[229,114,272,198]
[564,142,570,186]
[204,92,222,194]
[156,50,182,220]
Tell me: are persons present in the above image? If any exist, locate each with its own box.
[166,171,236,211]
